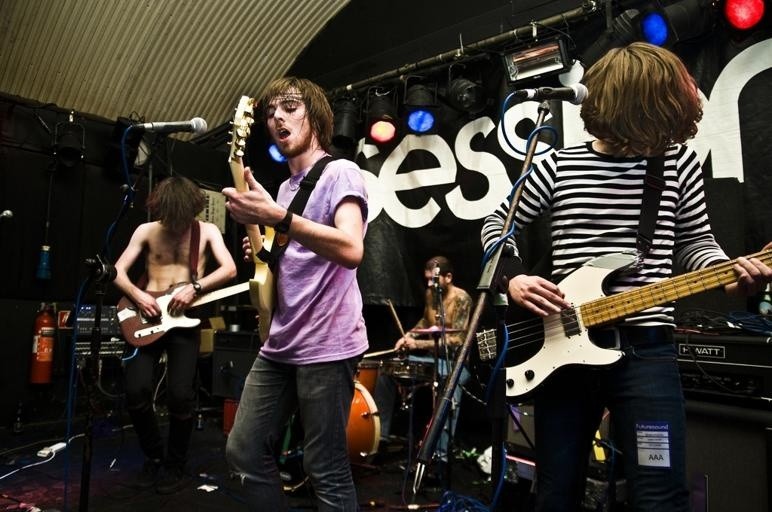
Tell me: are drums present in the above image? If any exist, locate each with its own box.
[354,361,383,396]
[345,379,380,466]
[387,360,438,382]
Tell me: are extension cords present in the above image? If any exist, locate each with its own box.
[38,441,67,458]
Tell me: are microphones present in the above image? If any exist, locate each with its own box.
[131,118,206,135]
[85,254,118,283]
[434,262,441,289]
[513,82,589,106]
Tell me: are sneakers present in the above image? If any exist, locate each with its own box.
[135,457,161,488]
[156,469,187,494]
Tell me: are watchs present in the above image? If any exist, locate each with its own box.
[192,279,202,297]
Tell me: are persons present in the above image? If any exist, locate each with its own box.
[394,257,473,359]
[480,41,768,512]
[112,176,236,494]
[222,76,369,511]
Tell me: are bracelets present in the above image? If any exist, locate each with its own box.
[274,209,293,235]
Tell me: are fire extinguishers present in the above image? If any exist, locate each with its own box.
[32,302,57,384]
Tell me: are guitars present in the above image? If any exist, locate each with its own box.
[117,281,255,347]
[468,248,772,403]
[227,95,276,343]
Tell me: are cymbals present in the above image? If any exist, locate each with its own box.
[408,324,465,338]
